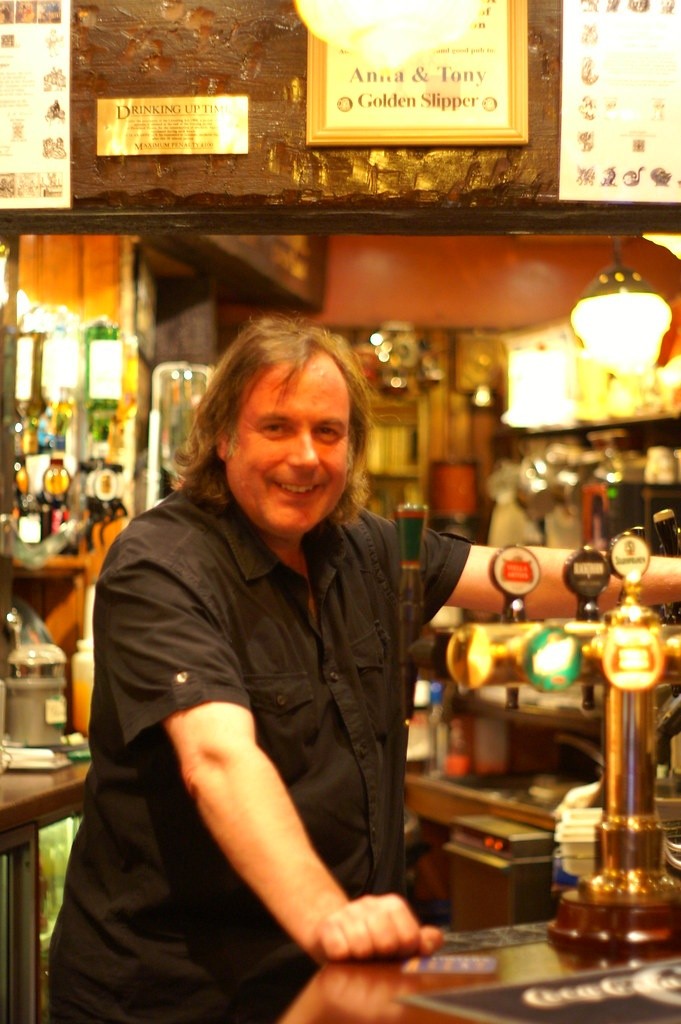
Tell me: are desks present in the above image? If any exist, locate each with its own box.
[405,765,602,834]
[0,752,90,1024]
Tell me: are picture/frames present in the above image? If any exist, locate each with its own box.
[305,0,527,148]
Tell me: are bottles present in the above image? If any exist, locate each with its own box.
[14,323,129,553]
[71,639,95,736]
[8,644,66,747]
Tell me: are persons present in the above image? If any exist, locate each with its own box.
[45,316,681,1023]
